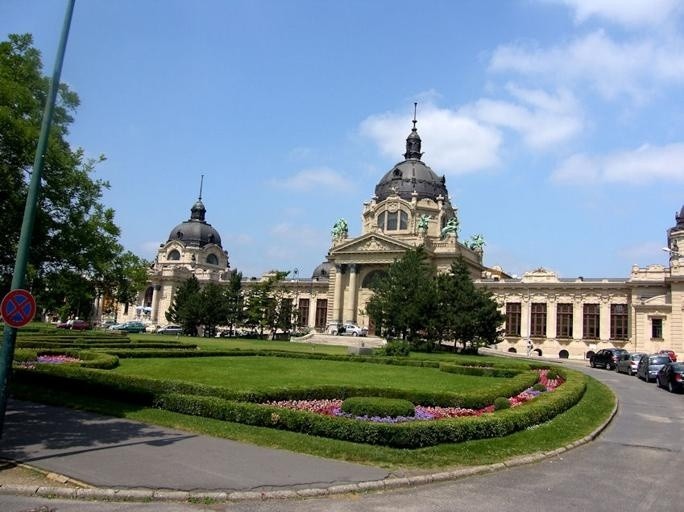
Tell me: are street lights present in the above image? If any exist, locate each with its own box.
[662,247,684,258]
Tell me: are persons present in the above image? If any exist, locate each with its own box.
[527,341,533,357]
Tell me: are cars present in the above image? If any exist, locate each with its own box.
[53,314,244,342]
[589,347,684,393]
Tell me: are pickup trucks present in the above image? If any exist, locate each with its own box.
[327,321,369,338]
